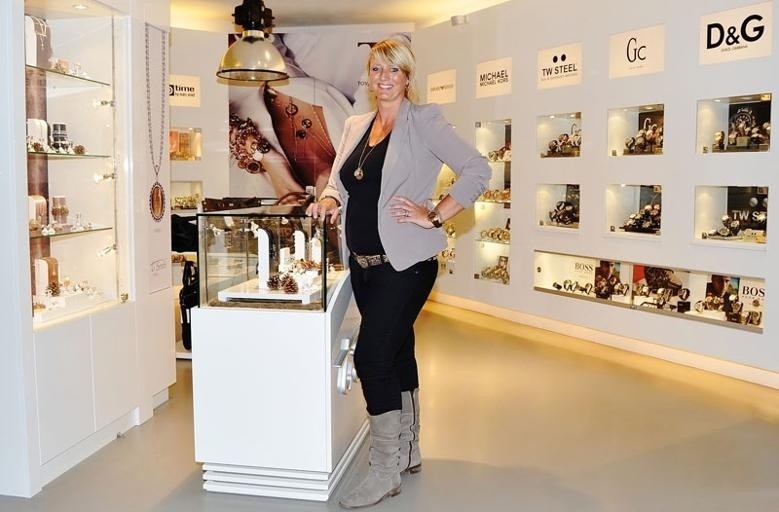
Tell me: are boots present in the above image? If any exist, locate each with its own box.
[339,407,403,510]
[397,387,422,475]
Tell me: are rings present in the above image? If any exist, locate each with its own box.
[403,209,410,217]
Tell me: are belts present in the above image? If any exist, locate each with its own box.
[350,250,390,269]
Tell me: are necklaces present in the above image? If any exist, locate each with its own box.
[31,14,48,52]
[353,117,393,181]
[269,93,336,178]
[47,259,58,278]
[145,24,166,223]
[37,195,45,224]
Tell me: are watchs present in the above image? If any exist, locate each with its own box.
[427,212,441,229]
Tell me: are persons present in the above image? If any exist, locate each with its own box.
[594,260,620,286]
[228,34,379,257]
[704,274,738,304]
[305,37,493,509]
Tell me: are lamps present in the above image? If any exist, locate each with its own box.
[215,1,291,87]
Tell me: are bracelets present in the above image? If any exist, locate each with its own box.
[229,112,270,174]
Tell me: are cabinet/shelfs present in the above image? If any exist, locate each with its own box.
[411,0,778,397]
[0,0,203,502]
